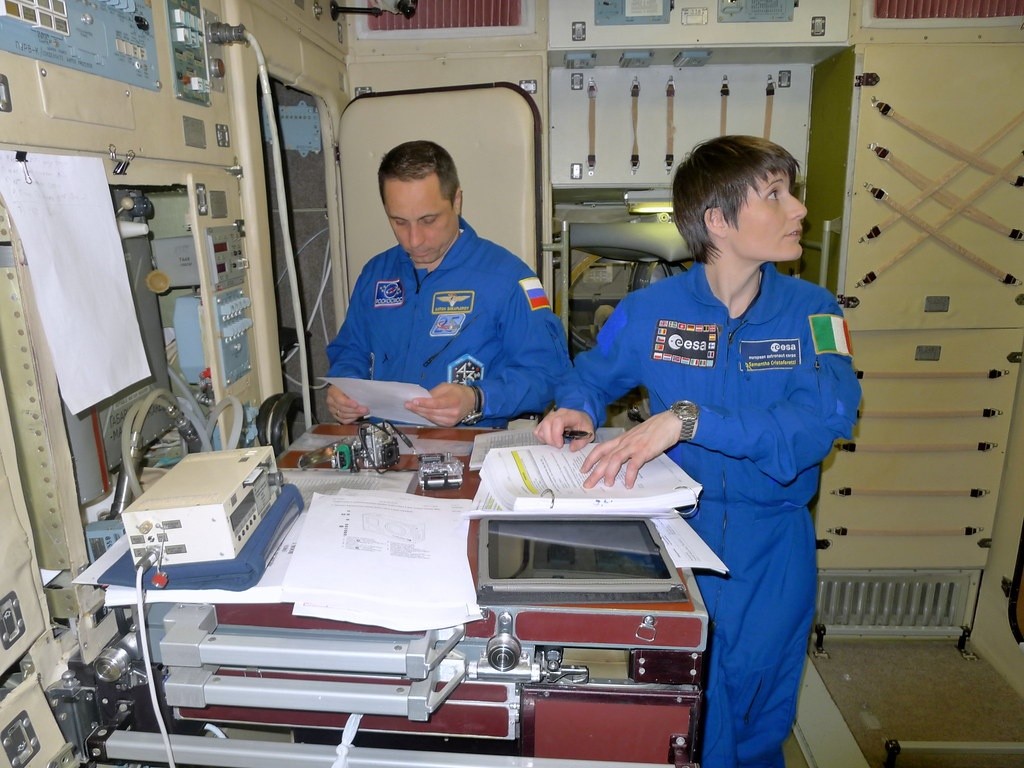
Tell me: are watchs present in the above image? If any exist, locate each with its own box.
[462,385,483,425]
[672,401,699,441]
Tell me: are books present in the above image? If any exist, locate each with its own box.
[479,443,702,511]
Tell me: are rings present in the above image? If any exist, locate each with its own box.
[336,410,340,417]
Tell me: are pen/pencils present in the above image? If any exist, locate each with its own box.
[564,430,590,440]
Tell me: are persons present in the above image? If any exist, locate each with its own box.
[326,141,572,429]
[533,134,862,768]
